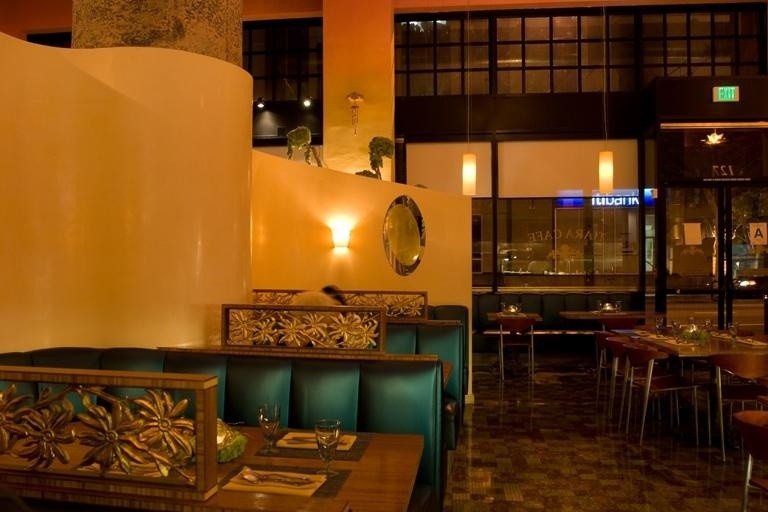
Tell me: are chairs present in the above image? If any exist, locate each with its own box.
[714,351,768,511]
[598,316,636,372]
[594,329,655,413]
[624,341,699,445]
[605,335,675,427]
[499,315,537,381]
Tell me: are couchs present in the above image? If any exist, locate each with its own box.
[250,318,464,450]
[420,307,471,428]
[127,346,446,512]
[473,292,631,355]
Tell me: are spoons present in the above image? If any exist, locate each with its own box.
[240,471,313,485]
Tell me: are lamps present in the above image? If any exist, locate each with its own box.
[304,95,312,107]
[701,130,724,145]
[598,151,614,195]
[326,214,358,259]
[461,151,476,197]
[257,96,264,113]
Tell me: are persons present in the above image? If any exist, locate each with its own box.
[526,247,553,274]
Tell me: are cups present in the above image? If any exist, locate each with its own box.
[705,319,711,335]
[728,322,738,345]
[655,316,664,338]
[316,421,340,478]
[258,402,280,457]
[672,319,681,339]
